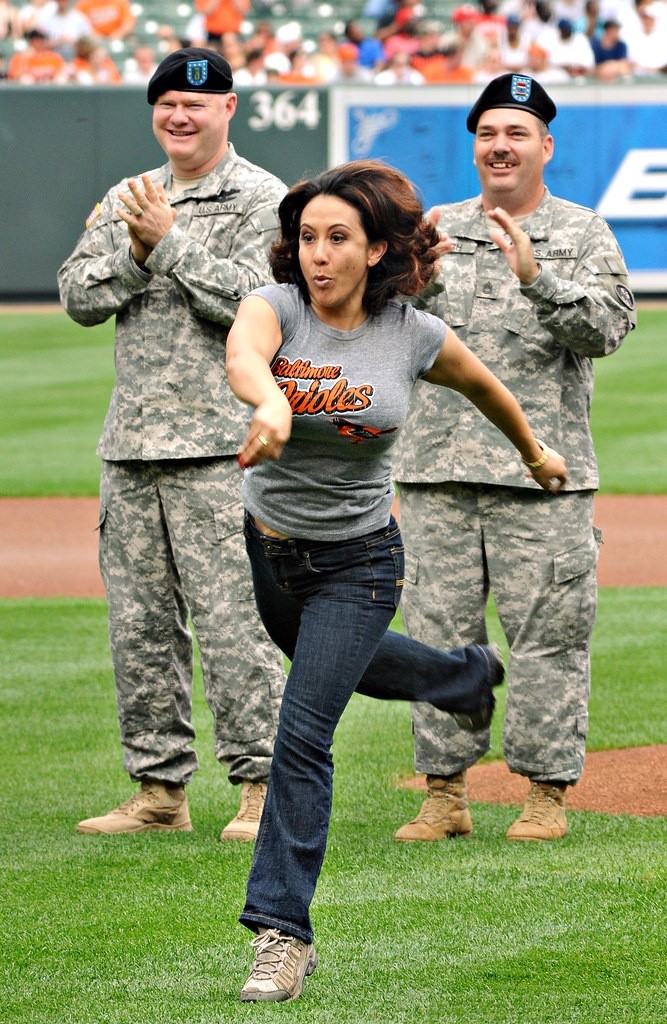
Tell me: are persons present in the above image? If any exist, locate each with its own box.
[390,72,637,842]
[53,47,289,841]
[0,0,667,95]
[224,160,568,1003]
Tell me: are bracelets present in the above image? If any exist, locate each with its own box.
[521,438,548,469]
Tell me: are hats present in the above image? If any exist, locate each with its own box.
[466,74,556,134]
[147,48,233,105]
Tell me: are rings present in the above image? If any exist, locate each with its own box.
[133,208,144,216]
[257,434,268,445]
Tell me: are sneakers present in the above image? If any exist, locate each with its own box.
[506,781,567,843]
[76,781,194,838]
[452,641,506,734]
[395,776,473,843]
[240,927,317,1004]
[220,779,267,841]
